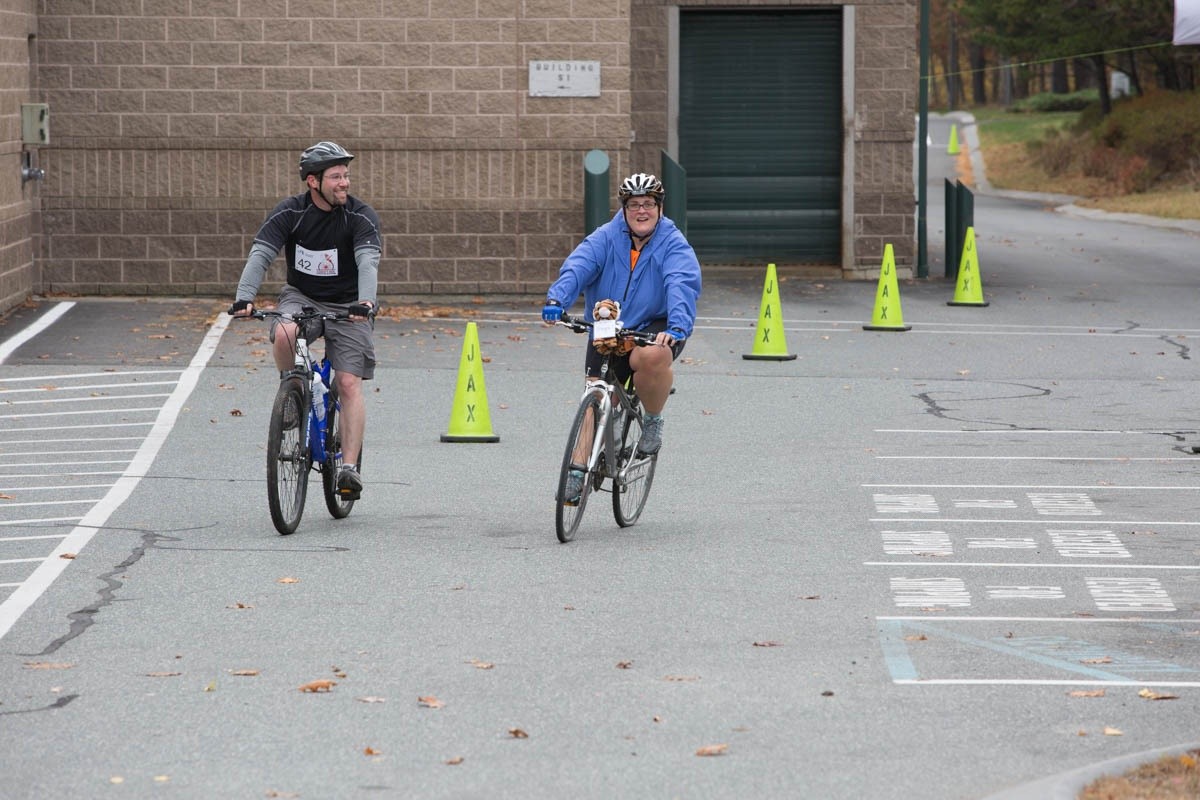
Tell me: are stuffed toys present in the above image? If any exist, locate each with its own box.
[592,299,637,355]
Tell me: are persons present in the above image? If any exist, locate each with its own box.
[233,142,382,501]
[542,172,701,507]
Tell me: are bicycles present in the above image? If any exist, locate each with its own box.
[544,309,685,546]
[228,301,381,537]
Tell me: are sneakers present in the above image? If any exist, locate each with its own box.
[283,395,300,432]
[638,411,663,454]
[554,466,591,502]
[337,468,363,502]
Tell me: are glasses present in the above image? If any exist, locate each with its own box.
[317,173,351,183]
[622,200,658,210]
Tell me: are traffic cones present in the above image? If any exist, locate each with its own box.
[739,261,801,361]
[436,321,501,445]
[942,226,994,309]
[860,240,915,333]
[946,121,962,157]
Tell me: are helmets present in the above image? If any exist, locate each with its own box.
[299,141,354,181]
[617,171,665,202]
